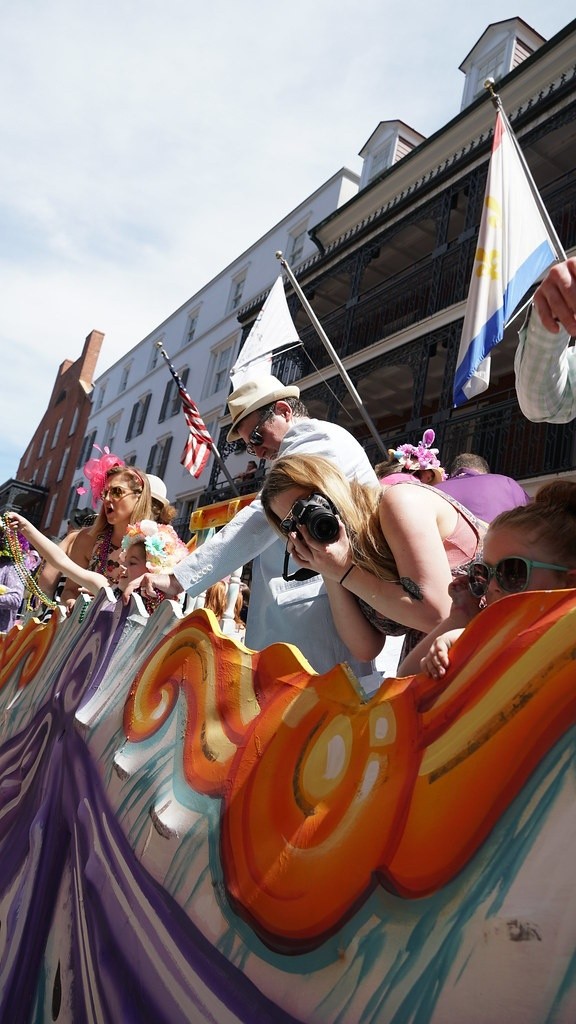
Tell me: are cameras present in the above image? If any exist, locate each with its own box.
[280,492,339,546]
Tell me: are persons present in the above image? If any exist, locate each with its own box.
[393,480,576,678]
[434,454,528,525]
[235,461,257,481]
[377,445,443,487]
[121,375,391,676]
[514,257,576,424]
[0,463,249,643]
[262,452,491,673]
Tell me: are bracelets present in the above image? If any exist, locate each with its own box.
[339,564,356,584]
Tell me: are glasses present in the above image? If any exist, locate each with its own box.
[246,403,275,456]
[67,520,71,525]
[467,556,570,599]
[99,486,136,501]
[279,495,312,536]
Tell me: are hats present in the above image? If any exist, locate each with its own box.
[145,474,170,508]
[70,508,98,529]
[225,374,300,443]
[388,428,449,486]
[119,520,189,575]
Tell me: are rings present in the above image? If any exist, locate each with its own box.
[141,587,146,590]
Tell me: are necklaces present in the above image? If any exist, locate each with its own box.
[3,512,57,610]
[146,588,179,614]
[27,558,42,612]
[96,528,119,584]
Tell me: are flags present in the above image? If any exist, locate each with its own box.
[169,363,213,477]
[452,111,557,405]
[230,275,301,388]
